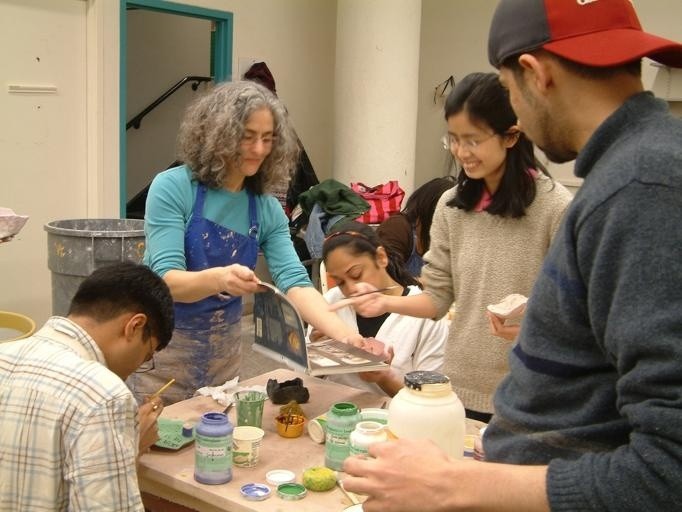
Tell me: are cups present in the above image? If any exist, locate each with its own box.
[231,426,265,469]
[232,390,265,428]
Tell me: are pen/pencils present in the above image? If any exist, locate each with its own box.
[151,379,174,399]
[343,286,399,299]
[223,402,232,412]
[285,408,291,431]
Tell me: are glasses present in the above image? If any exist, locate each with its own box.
[132,323,156,374]
[444,129,497,148]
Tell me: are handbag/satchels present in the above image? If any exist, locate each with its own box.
[350,181,404,224]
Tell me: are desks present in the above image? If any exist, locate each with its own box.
[134,369,487,512]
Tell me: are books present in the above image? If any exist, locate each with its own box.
[251,282,390,376]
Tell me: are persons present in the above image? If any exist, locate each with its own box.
[0,261,175,512]
[376,176,457,278]
[342,0,682,512]
[305,220,448,399]
[328,73,575,423]
[125,81,364,407]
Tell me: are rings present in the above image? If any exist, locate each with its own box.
[151,401,158,411]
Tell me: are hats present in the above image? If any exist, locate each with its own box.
[487,0,681,69]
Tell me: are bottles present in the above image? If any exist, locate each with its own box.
[193,411,235,487]
[473,427,487,462]
[307,370,467,472]
